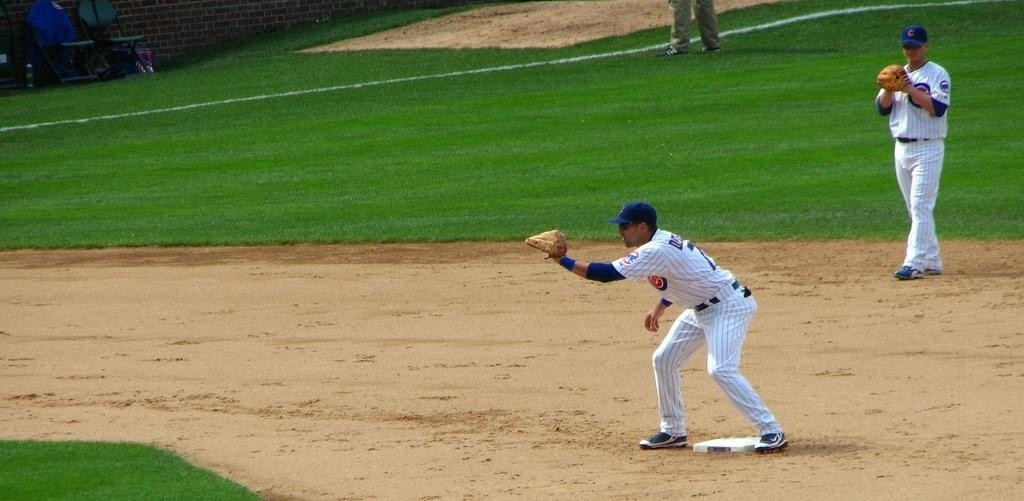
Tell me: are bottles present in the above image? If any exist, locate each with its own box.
[25,63,34,87]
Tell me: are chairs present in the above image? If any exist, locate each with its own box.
[26,0,100,86]
[78,0,149,76]
[0,0,24,89]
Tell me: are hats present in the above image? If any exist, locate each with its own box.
[902,25,927,46]
[610,202,657,223]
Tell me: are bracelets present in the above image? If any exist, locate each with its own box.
[560,257,576,271]
[660,298,672,307]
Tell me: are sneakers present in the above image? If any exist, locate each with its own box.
[894,265,940,280]
[657,47,688,56]
[754,432,788,451]
[639,432,686,448]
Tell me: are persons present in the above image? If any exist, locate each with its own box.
[526,202,791,454]
[655,0,720,58]
[872,25,950,281]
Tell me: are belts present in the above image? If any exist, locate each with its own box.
[694,281,739,311]
[897,137,929,143]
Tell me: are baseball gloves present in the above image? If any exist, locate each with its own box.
[526,230,566,259]
[876,64,912,92]
